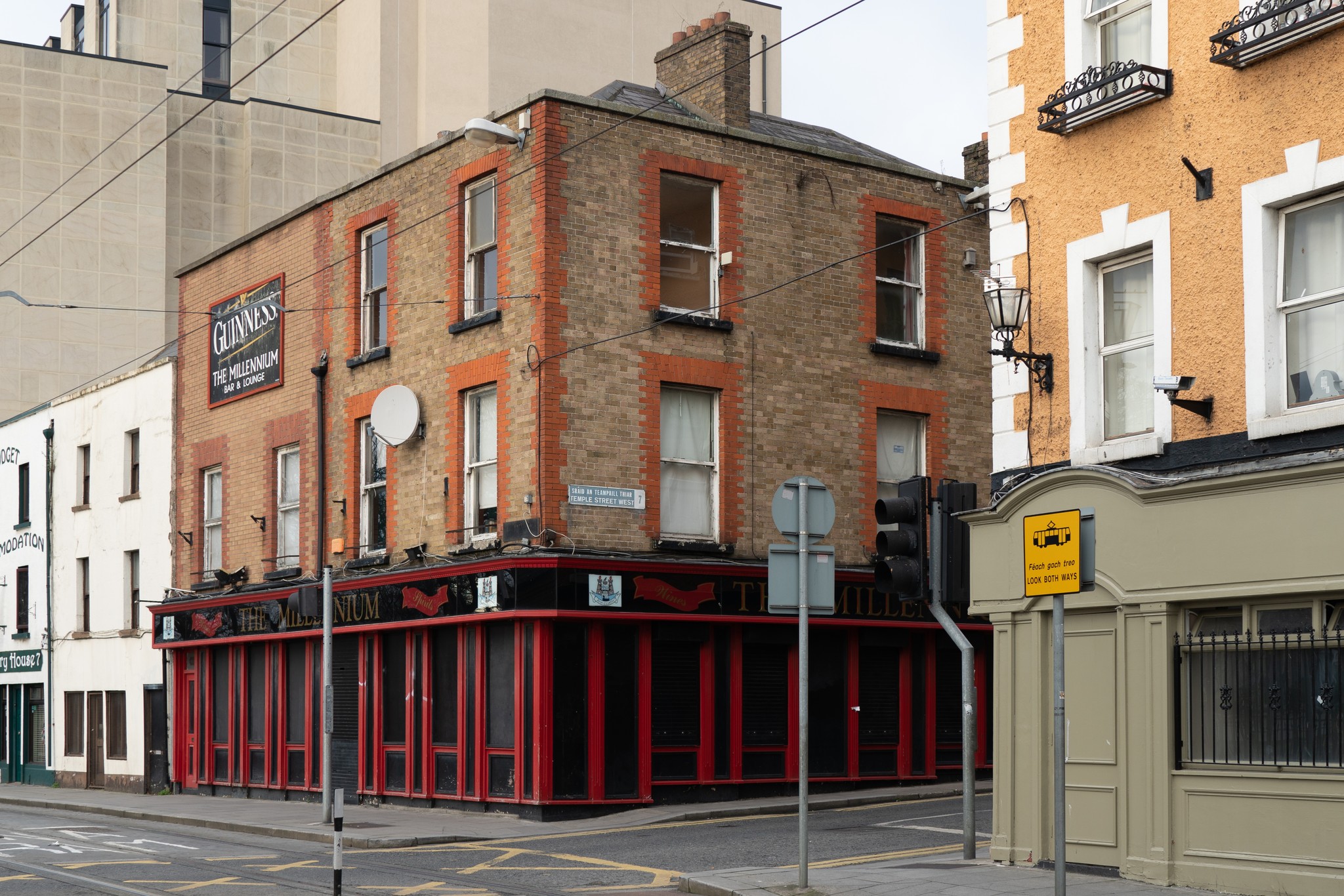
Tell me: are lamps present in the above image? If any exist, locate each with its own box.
[461,110,533,155]
[967,260,1056,399]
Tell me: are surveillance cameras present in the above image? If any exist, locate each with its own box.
[1153,375,1197,392]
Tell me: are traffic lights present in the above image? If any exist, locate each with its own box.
[875,476,929,602]
